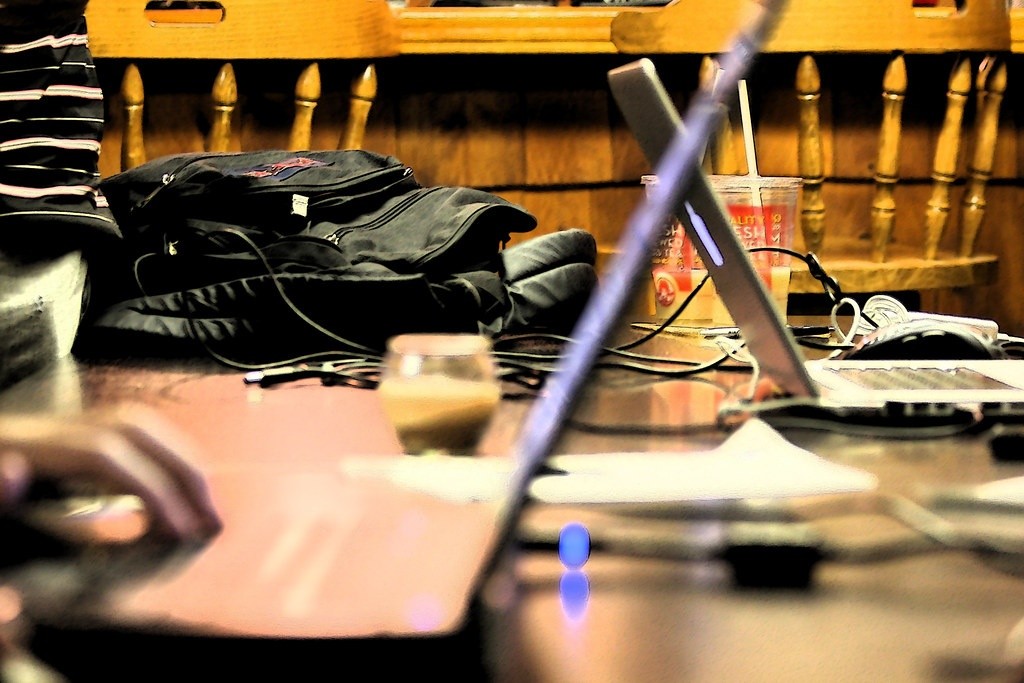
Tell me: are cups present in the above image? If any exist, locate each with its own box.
[382,331,497,456]
[640,172,800,340]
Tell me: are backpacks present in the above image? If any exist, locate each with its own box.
[68,150,600,364]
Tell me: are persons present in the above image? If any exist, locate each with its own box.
[0,0,123,389]
[0,398,225,546]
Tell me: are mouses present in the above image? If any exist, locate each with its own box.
[843,319,997,359]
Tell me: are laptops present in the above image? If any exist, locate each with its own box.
[607,59,1024,425]
[0,1,790,674]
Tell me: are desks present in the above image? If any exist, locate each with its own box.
[1,316,1024,683]
